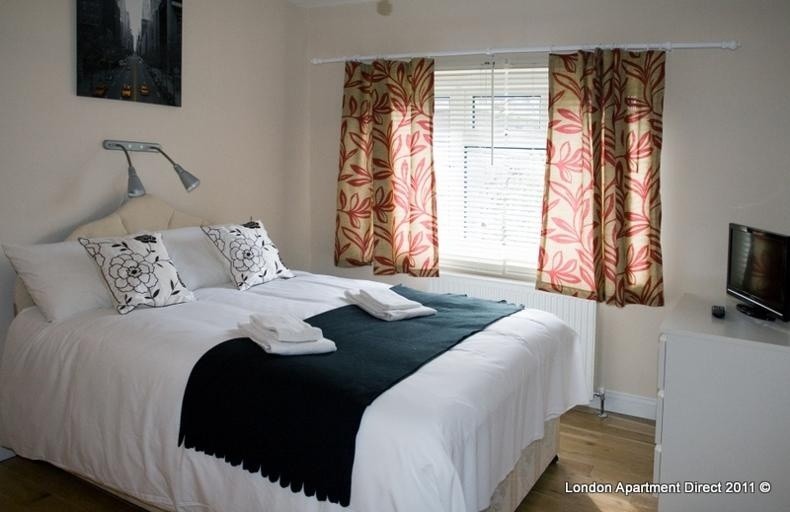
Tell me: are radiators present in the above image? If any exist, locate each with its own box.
[383,272,613,422]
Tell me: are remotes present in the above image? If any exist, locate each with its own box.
[711,305,726,319]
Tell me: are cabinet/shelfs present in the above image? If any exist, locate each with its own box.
[651,292,790,510]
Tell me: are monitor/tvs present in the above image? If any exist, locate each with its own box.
[726,223,790,323]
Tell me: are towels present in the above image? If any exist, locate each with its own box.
[344,283,438,322]
[237,311,338,357]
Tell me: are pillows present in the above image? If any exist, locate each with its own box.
[146,226,237,289]
[200,218,297,292]
[9,240,113,325]
[76,230,197,316]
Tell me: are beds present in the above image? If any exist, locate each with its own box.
[1,195,584,512]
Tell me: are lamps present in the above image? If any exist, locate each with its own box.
[103,139,200,198]
